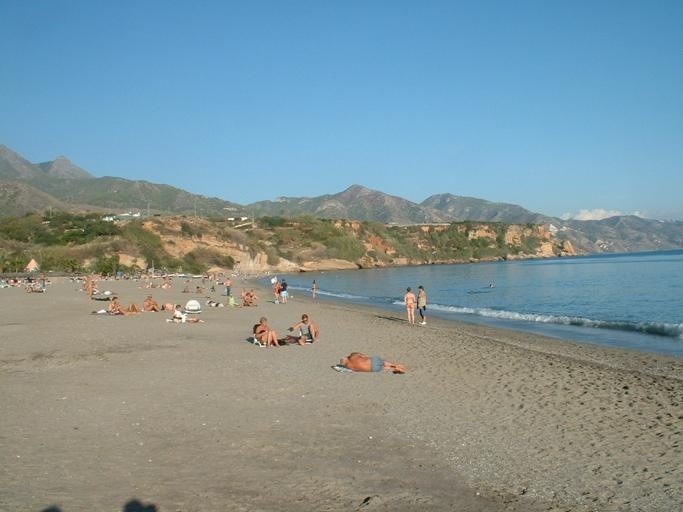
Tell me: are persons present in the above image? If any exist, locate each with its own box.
[404,287,416,324]
[255,317,280,349]
[416,285,427,325]
[339,351,405,374]
[0,267,51,295]
[310,280,319,298]
[282,313,319,346]
[67,267,289,326]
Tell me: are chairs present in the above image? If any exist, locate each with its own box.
[300,330,314,344]
[253,333,273,348]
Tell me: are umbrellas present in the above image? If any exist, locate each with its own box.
[25,257,40,278]
